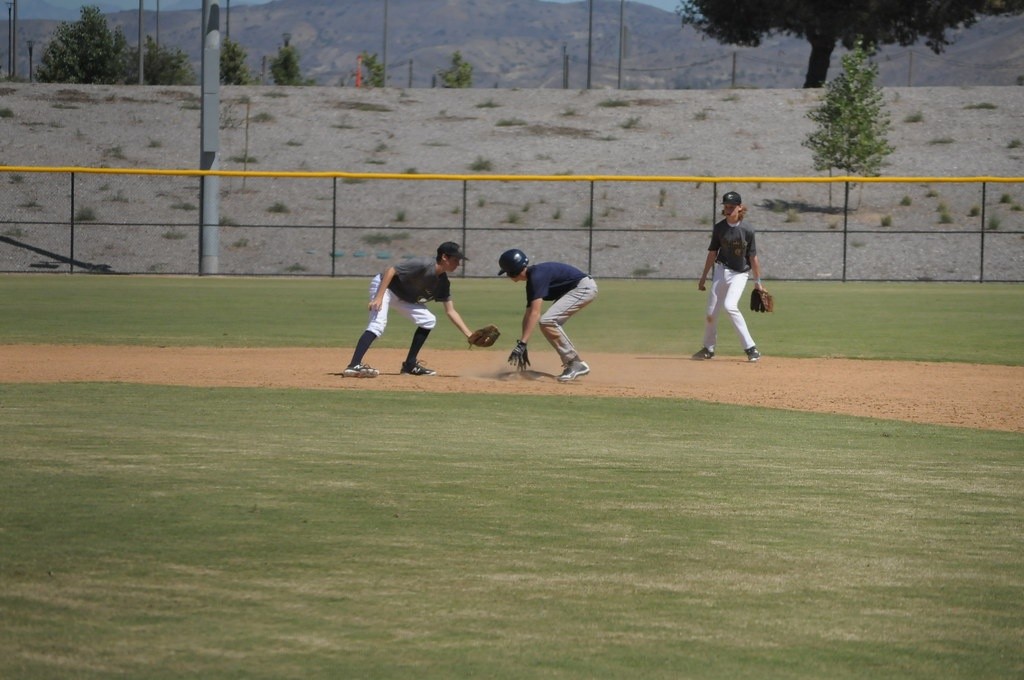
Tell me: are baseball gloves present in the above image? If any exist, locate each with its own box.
[468,324,501,350]
[750,285,773,313]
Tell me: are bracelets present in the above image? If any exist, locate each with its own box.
[756,279,761,284]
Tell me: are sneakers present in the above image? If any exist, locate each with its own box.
[343,364,378,377]
[557,361,590,381]
[745,347,761,361]
[400,360,436,376]
[692,347,715,359]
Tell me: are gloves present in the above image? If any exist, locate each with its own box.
[508,339,530,372]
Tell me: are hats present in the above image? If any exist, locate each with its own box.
[720,192,741,206]
[440,241,468,260]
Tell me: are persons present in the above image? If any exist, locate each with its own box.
[343,240,500,378]
[692,192,767,362]
[497,249,599,382]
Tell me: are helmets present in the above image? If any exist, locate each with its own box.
[498,249,529,277]
